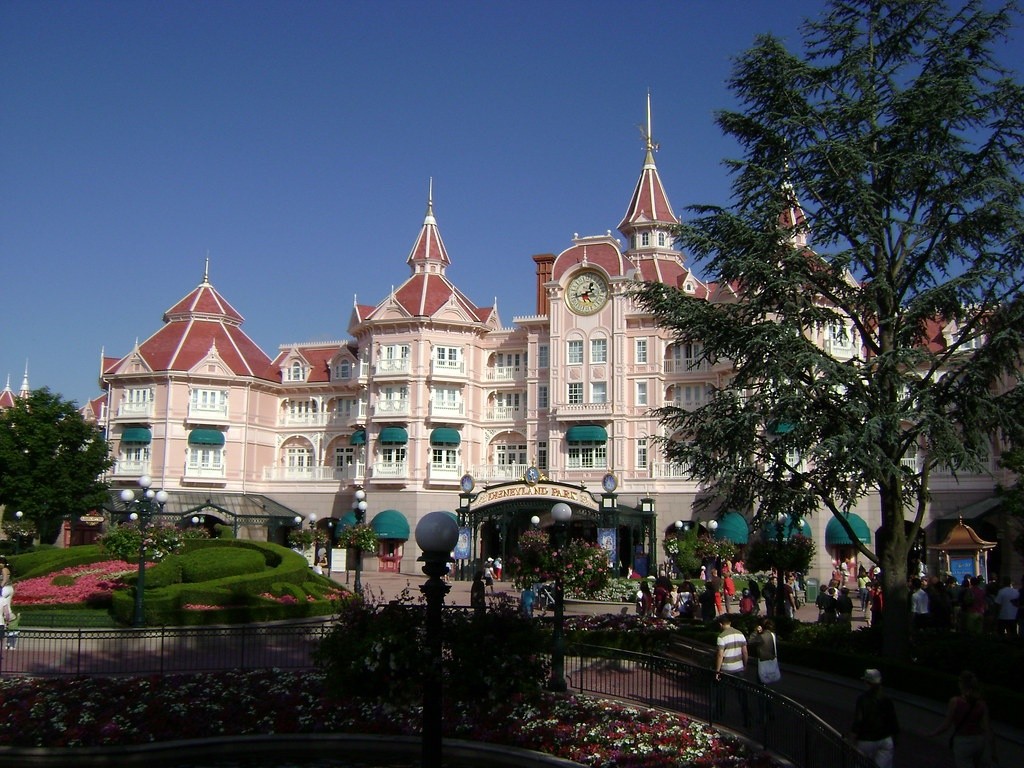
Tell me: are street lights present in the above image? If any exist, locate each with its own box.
[641,497,659,579]
[548,503,572,693]
[294,513,333,578]
[351,484,368,593]
[411,510,460,768]
[120,476,169,630]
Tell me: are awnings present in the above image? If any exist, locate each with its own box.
[349,427,460,445]
[121,426,224,445]
[566,425,608,442]
[334,511,462,541]
[711,414,872,544]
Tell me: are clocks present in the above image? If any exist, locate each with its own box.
[564,269,610,317]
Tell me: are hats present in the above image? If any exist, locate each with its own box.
[860,669,881,683]
[701,566,705,569]
[488,558,494,561]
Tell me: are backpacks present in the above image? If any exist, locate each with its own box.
[679,592,694,612]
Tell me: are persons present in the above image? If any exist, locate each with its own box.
[627,555,806,620]
[857,566,883,629]
[748,617,778,721]
[921,671,1002,768]
[711,613,754,729]
[0,557,17,659]
[906,552,1024,637]
[314,547,329,577]
[482,556,503,593]
[470,571,486,615]
[847,667,901,768]
[520,578,535,619]
[815,579,854,622]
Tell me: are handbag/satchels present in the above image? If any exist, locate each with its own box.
[5,631,19,650]
[757,658,781,683]
